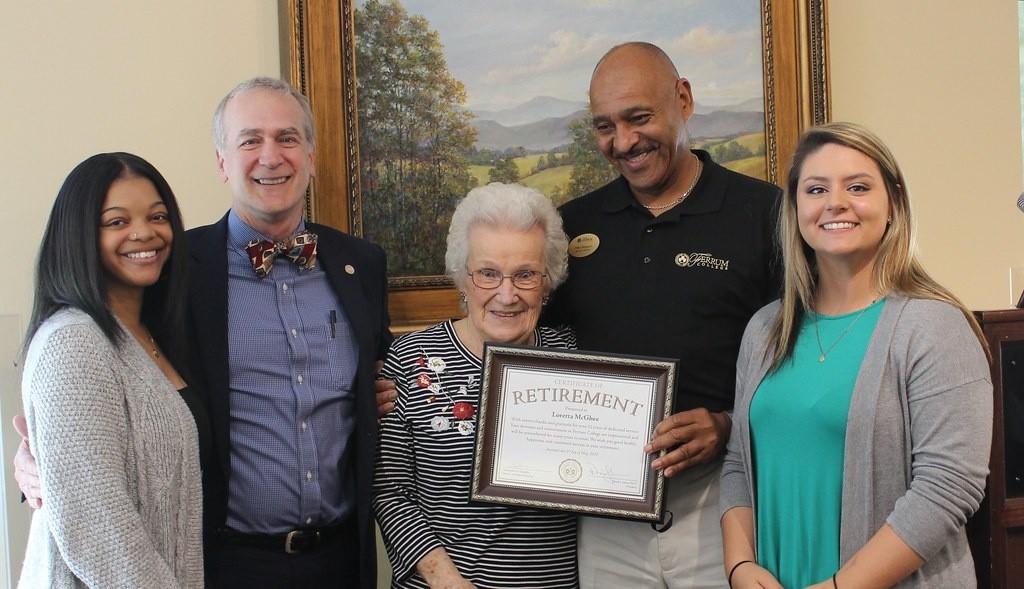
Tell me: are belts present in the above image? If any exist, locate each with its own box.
[220,518,353,556]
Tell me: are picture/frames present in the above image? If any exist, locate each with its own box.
[288,0,831,340]
[466,344,680,527]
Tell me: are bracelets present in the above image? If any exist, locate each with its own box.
[833,573,838,589]
[718,123,994,589]
[728,560,752,589]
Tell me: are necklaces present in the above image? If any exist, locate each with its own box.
[131,322,159,356]
[811,291,878,362]
[644,152,699,209]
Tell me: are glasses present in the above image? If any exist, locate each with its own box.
[464,265,547,290]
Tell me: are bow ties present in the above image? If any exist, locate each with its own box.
[243,229,318,279]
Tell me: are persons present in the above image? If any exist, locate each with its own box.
[374,41,785,589]
[16,152,206,589]
[364,183,586,589]
[13,77,394,589]
